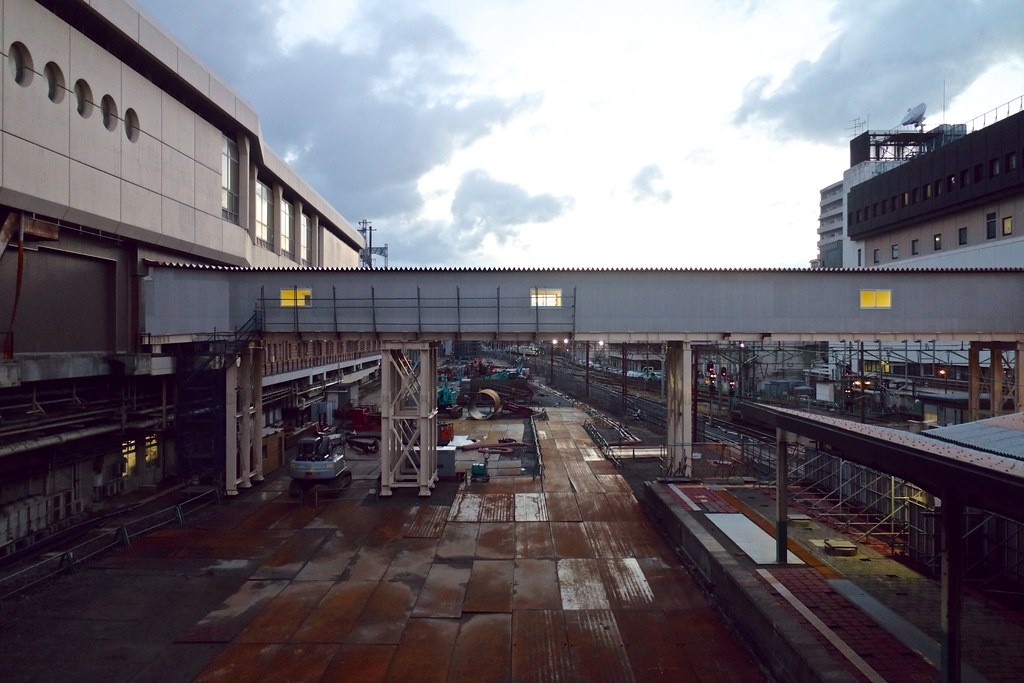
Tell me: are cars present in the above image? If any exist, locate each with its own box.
[578,359,662,383]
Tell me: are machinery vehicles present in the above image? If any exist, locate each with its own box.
[332,399,456,453]
[286,423,359,500]
[437,373,463,419]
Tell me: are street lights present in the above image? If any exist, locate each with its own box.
[873,339,883,383]
[914,339,922,376]
[839,337,846,376]
[901,339,908,393]
[939,368,948,427]
[854,339,861,379]
[928,339,936,378]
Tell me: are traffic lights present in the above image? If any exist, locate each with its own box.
[721,366,727,377]
[843,369,851,377]
[729,380,735,389]
[710,373,717,386]
[706,361,714,373]
[844,388,852,394]
